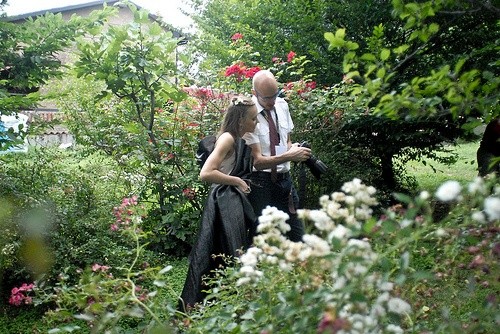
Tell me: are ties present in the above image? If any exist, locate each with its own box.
[265,109,279,183]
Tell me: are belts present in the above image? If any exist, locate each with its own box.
[252,171,290,180]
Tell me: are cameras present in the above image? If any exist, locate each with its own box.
[300,141,328,180]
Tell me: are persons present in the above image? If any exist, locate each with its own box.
[241,69,313,244]
[173,93,262,320]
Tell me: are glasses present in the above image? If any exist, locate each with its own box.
[255,91,279,102]
